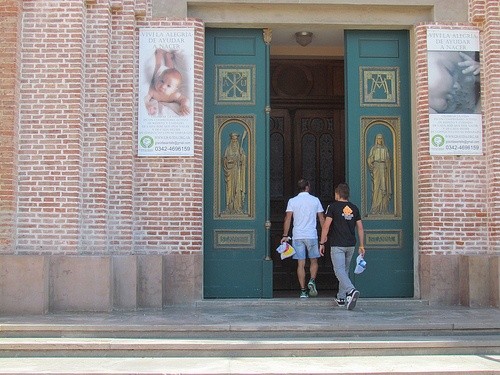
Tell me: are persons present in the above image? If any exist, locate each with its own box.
[281,178,327,298]
[368,134,392,214]
[223,133,246,214]
[144,49,190,116]
[428,50,480,115]
[319,184,365,310]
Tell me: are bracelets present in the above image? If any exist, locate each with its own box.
[320,243,324,245]
[283,236,288,237]
[359,247,364,249]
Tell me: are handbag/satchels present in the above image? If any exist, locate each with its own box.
[280,242,297,260]
[353,254,367,274]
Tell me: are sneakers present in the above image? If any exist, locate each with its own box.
[300,289,307,297]
[308,279,318,297]
[346,288,360,310]
[336,298,345,307]
